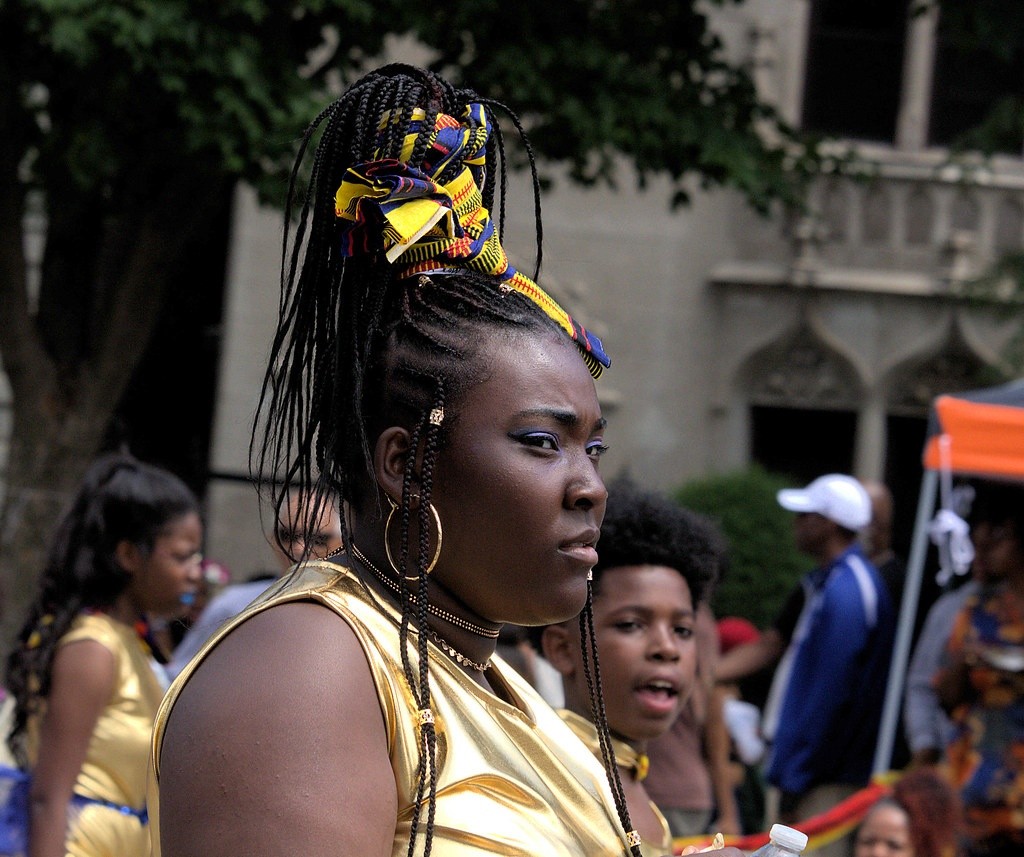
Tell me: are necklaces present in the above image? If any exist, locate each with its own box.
[316,538,499,672]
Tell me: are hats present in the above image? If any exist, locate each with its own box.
[778,474,872,529]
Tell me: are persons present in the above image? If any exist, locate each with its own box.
[6,64,1024,857]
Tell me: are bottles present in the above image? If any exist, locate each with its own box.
[747,823,809,857]
